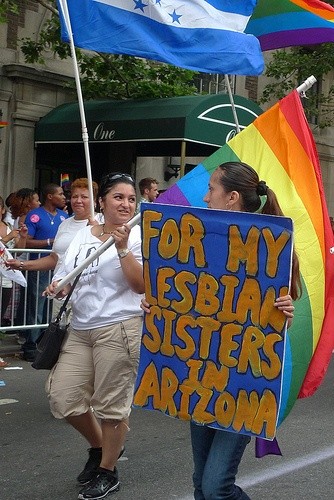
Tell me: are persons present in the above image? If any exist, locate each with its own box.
[45,172,145,500]
[136,177,159,214]
[140,161,303,500]
[0,188,41,334]
[4,178,105,326]
[24,184,71,343]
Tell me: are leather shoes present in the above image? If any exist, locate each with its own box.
[24,351,36,362]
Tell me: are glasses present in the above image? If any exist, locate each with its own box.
[105,172,136,184]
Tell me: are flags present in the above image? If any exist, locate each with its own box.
[153,90,334,458]
[58,0,265,75]
[244,0,334,52]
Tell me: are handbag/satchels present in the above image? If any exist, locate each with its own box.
[31,322,70,371]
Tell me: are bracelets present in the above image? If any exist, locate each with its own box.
[19,261,24,270]
[47,238,50,246]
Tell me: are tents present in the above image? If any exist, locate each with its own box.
[34,93,265,180]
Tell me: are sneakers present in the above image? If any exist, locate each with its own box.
[17,335,27,344]
[0,358,9,367]
[77,444,126,500]
[5,331,17,337]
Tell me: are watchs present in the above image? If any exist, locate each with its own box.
[117,249,130,258]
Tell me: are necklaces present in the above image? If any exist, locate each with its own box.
[43,206,57,225]
[98,223,115,237]
